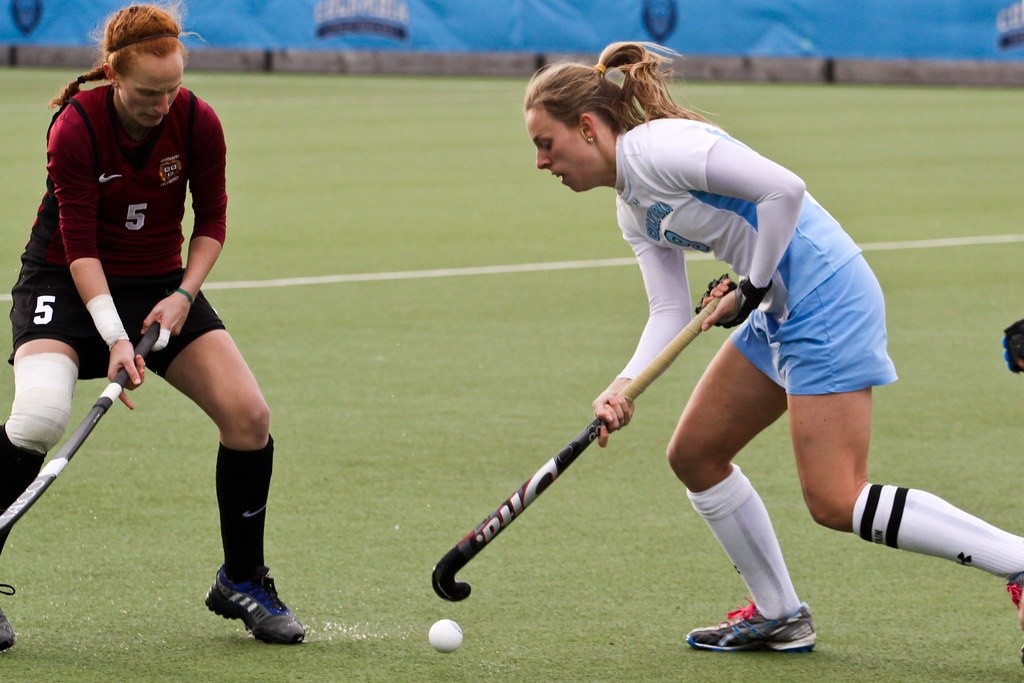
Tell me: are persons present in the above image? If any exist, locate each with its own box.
[0,5,305,646]
[524,40,1023,668]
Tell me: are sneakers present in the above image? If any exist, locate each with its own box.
[1007,572,1024,662]
[205,565,306,644]
[0,583,20,650]
[687,598,817,652]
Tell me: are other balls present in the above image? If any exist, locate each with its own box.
[428,619,463,652]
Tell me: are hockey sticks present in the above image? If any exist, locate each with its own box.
[430,294,720,602]
[0,320,160,552]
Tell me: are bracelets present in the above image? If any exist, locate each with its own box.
[166,287,193,305]
